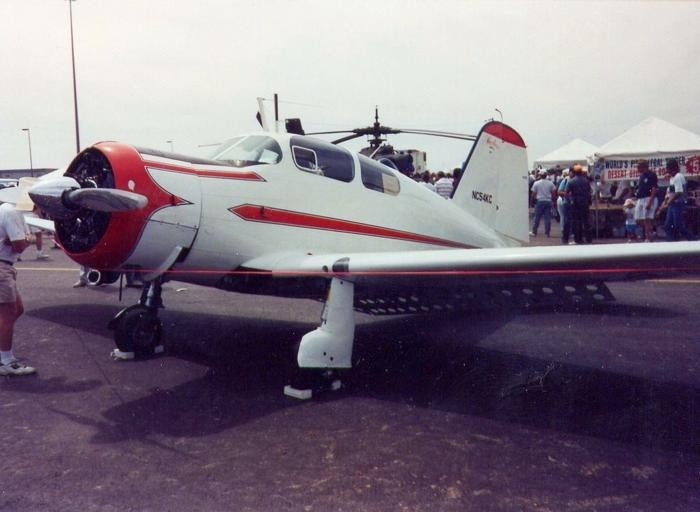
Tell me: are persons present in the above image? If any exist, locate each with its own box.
[656,161,694,242]
[0,203,37,377]
[409,169,463,198]
[529,158,636,245]
[16,204,50,261]
[73,266,92,288]
[631,159,660,243]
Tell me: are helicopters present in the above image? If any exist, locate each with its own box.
[302,104,479,176]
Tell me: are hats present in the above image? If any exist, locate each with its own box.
[624,199,632,206]
[573,164,581,172]
[539,169,546,175]
[639,160,649,168]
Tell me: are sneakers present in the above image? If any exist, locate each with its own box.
[529,232,536,237]
[0,359,35,375]
[569,241,577,244]
[73,279,85,287]
[38,254,49,259]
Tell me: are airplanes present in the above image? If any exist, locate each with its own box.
[22,116,699,403]
